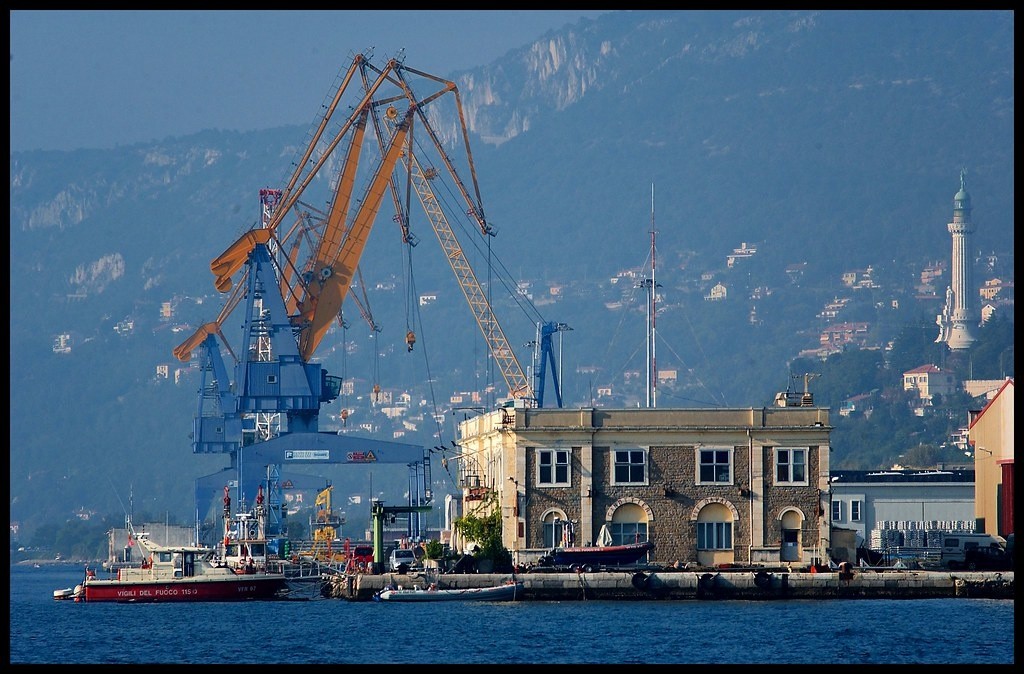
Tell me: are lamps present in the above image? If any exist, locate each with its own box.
[662,488,667,498]
[738,488,743,499]
[587,489,591,497]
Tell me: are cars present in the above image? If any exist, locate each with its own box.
[389,548,419,573]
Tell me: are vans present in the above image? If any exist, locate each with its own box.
[350,544,374,571]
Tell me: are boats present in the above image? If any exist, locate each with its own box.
[53,587,72,600]
[379,579,526,601]
[553,542,654,567]
[78,482,288,603]
[372,595,382,603]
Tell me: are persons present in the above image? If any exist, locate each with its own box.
[787,561,793,573]
[513,562,534,574]
[668,552,687,568]
[827,559,833,573]
